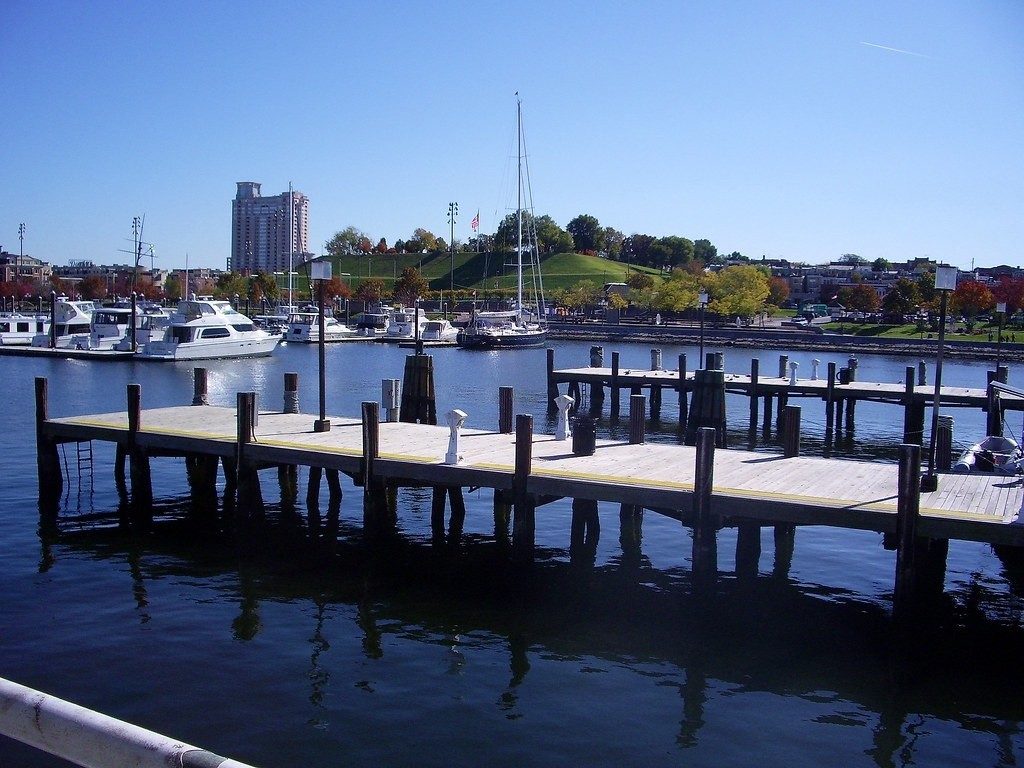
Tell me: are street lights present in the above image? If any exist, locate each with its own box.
[921,267,958,492]
[699,293,708,368]
[996,302,1006,380]
[311,260,333,432]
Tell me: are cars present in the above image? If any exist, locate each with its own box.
[849,312,883,321]
[595,310,605,316]
[793,310,827,321]
[904,314,965,323]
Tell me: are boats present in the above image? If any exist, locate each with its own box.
[251,306,426,340]
[954,436,1024,476]
[0,300,234,350]
[398,320,459,347]
[134,315,282,360]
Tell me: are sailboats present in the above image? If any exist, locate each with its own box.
[456,91,549,350]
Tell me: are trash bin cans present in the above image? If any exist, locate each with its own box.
[839,367,852,385]
[569,415,599,456]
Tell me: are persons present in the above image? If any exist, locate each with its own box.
[1011,333,1015,342]
[1006,335,1009,342]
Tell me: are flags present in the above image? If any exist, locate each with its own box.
[472,216,479,228]
